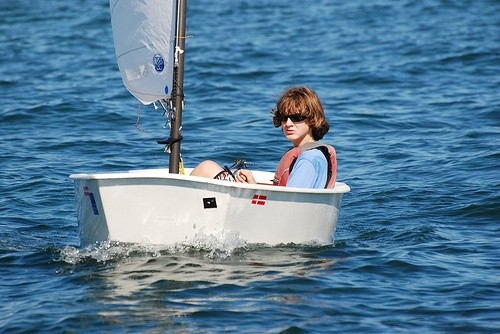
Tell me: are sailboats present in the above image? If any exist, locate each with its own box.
[67,0,350,256]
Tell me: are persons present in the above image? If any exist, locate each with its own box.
[189,86,337,189]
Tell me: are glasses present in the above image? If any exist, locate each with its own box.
[279,114,309,124]
